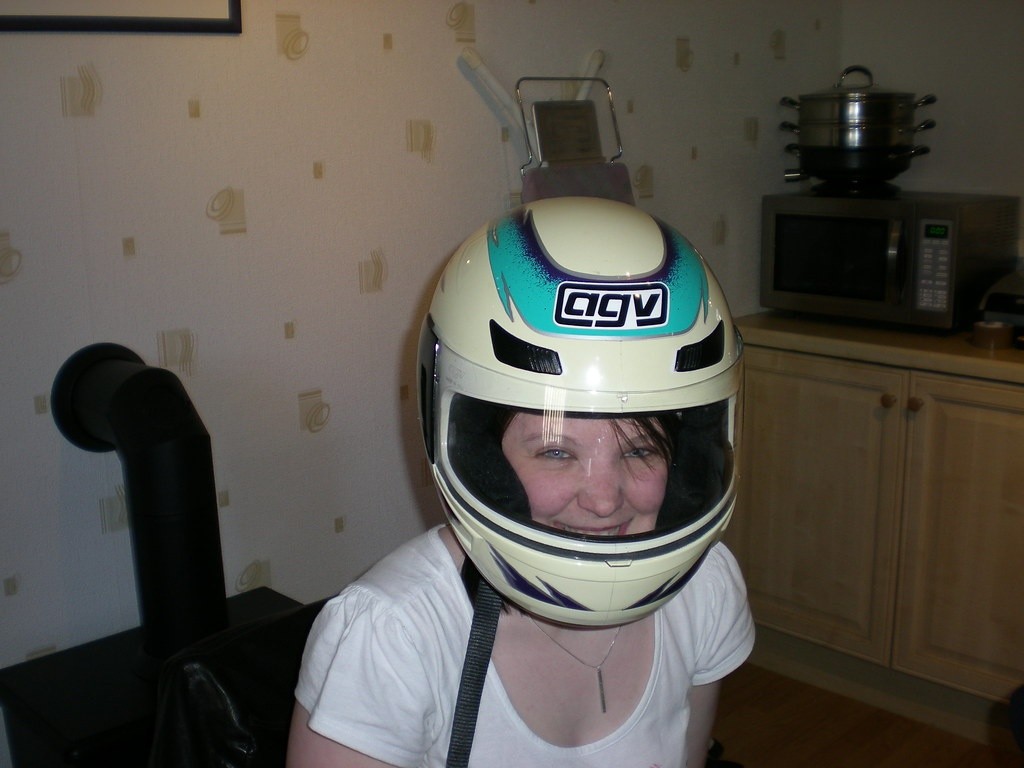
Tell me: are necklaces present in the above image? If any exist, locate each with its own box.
[528,615,620,712]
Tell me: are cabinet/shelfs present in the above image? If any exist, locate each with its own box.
[720,343,1024,755]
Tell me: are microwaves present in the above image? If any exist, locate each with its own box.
[762,190,1021,331]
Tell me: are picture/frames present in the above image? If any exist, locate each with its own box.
[0,0,242,35]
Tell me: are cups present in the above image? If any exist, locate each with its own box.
[968,321,1014,349]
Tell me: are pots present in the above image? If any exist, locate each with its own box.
[782,66,936,189]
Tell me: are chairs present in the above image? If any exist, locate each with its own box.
[148,601,323,768]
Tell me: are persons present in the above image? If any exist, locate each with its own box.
[287,197,754,768]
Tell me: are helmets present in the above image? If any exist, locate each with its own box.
[416,196,746,629]
